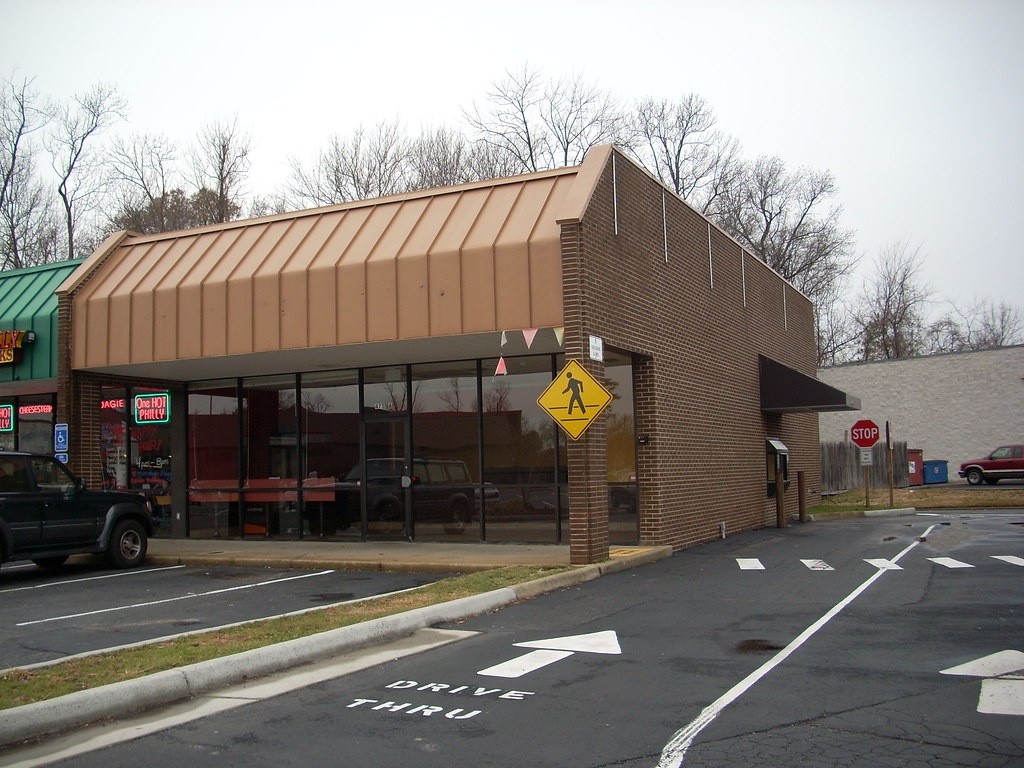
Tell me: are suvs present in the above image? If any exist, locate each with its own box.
[0,451,151,569]
[611,471,637,512]
[304,455,476,534]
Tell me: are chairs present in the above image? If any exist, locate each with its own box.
[157,496,172,533]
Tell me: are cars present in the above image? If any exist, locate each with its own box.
[475,482,500,502]
[958,444,1024,485]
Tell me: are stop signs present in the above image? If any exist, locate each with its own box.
[851,420,880,449]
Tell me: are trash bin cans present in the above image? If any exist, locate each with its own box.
[924,459,949,484]
[907,448,925,486]
